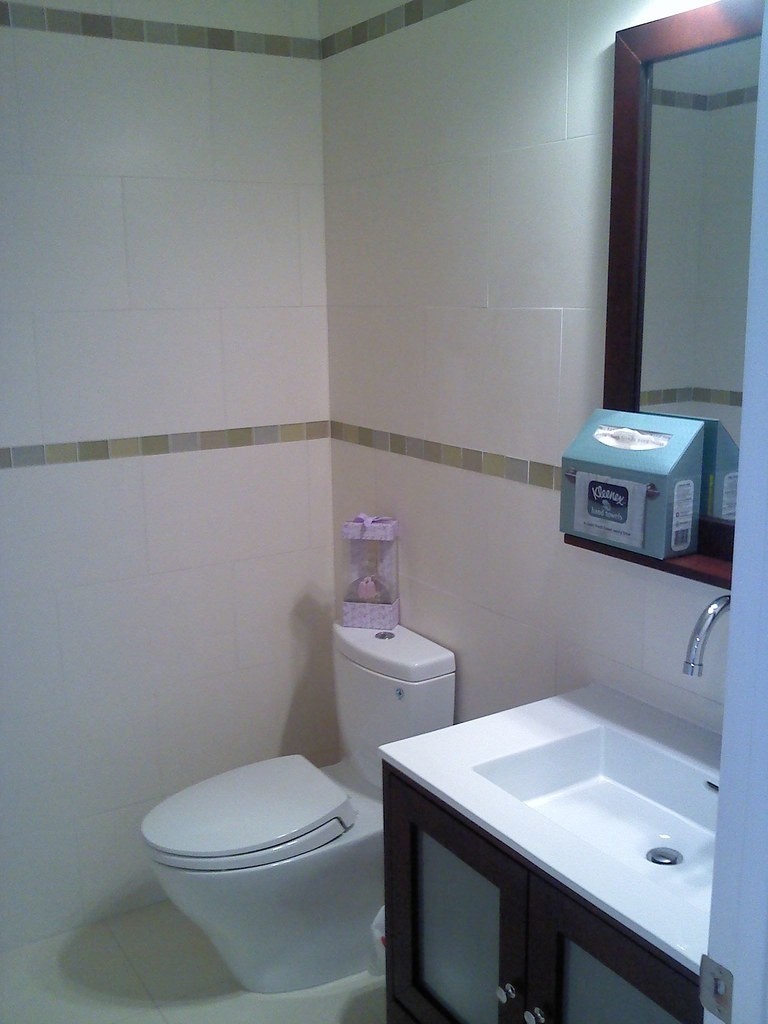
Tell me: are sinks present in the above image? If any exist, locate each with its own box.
[379,681,733,976]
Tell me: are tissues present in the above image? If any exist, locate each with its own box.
[554,408,709,561]
[638,414,738,522]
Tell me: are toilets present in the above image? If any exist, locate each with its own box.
[134,612,460,999]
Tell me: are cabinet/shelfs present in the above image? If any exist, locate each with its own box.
[381,758,705,1024]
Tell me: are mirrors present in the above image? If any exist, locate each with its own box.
[600,1,765,563]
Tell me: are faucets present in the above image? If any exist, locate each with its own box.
[679,588,733,679]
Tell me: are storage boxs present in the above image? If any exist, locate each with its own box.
[559,408,704,560]
[638,410,741,522]
